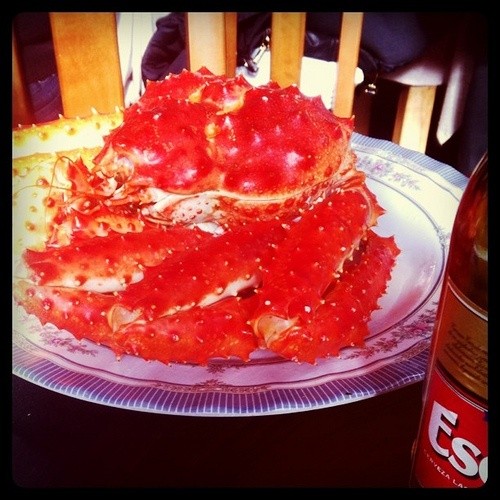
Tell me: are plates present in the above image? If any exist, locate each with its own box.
[12,111,472,418]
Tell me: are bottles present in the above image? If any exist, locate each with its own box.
[408,152,489,487]
